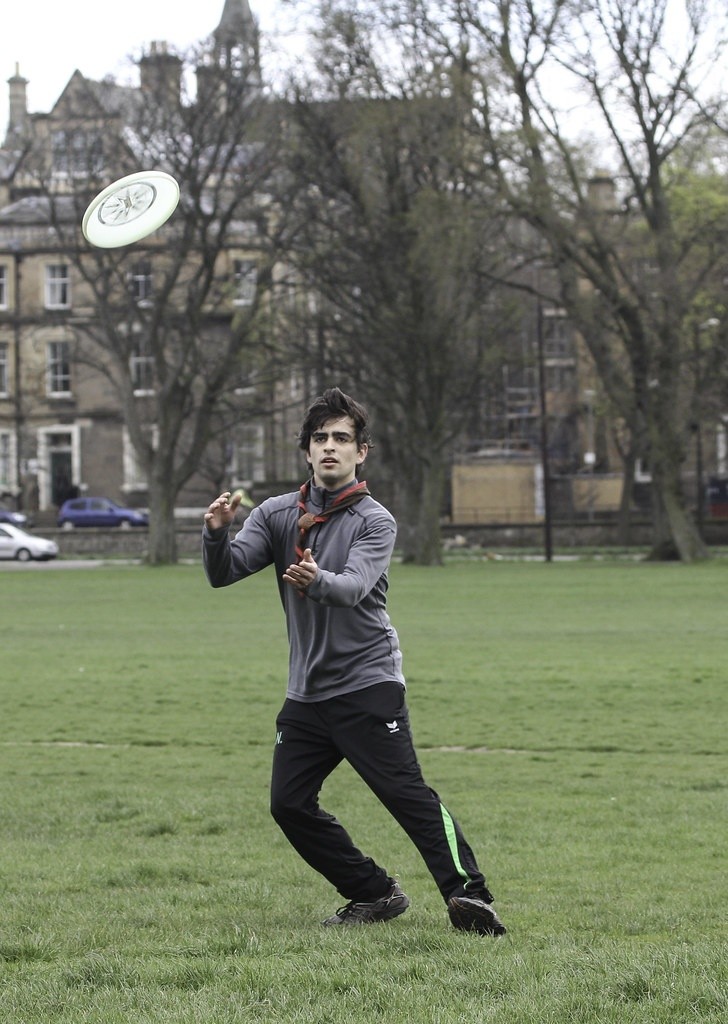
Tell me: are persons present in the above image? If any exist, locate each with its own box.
[198,384,508,937]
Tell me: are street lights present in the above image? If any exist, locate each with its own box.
[537,305,572,564]
[698,317,722,541]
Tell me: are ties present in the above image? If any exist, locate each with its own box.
[295,479,371,598]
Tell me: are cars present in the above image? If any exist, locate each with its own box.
[0,523,60,563]
[0,499,28,528]
[57,496,150,531]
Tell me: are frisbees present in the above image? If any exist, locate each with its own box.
[81,169,181,249]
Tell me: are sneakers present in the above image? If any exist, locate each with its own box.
[448,897,506,937]
[320,877,409,927]
